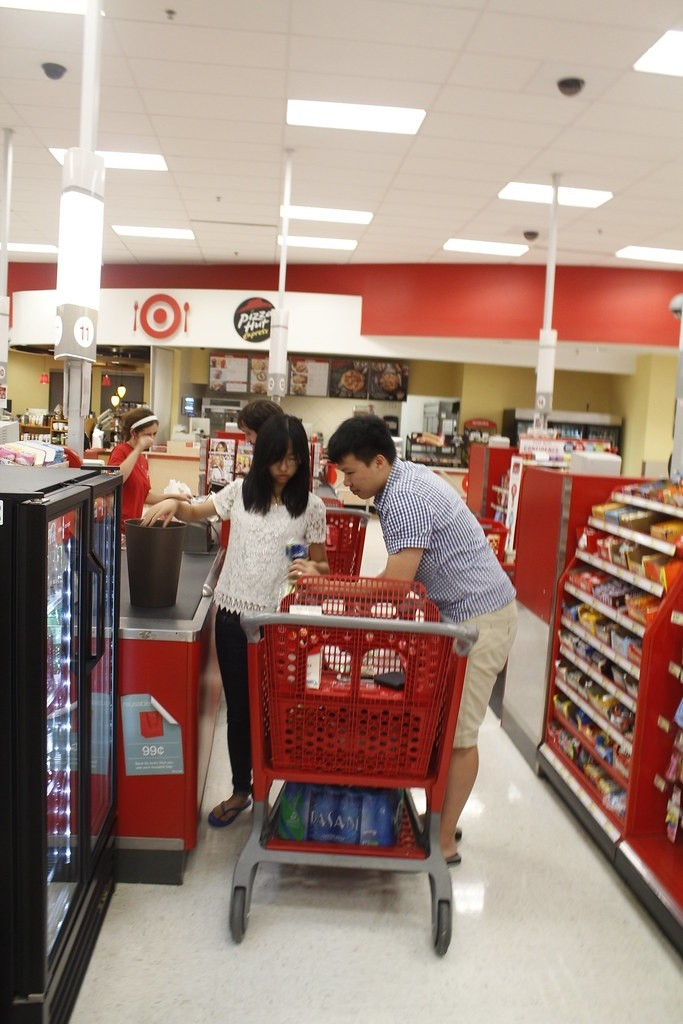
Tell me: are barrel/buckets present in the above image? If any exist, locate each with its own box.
[123,518,187,608]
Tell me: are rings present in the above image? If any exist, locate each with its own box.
[297,571,303,578]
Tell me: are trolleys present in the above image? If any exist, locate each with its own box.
[224,573,477,956]
[319,496,372,584]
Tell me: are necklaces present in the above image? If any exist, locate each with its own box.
[273,493,282,506]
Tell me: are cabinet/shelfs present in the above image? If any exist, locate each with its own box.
[0,399,254,495]
[462,409,683,961]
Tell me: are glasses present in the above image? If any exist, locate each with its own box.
[272,455,301,465]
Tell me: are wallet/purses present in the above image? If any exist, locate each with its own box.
[374,672,407,689]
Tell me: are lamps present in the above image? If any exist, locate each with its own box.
[110,367,120,406]
[117,368,127,398]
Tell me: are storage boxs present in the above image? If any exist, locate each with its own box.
[627,548,664,578]
[624,673,640,702]
[611,540,644,570]
[620,510,663,532]
[588,686,618,718]
[649,519,683,542]
[592,503,626,521]
[596,536,625,562]
[571,568,613,595]
[579,606,606,637]
[610,663,626,690]
[625,592,661,629]
[593,577,639,609]
[611,629,639,658]
[644,557,681,584]
[627,644,643,667]
[562,598,586,622]
[575,527,607,553]
[605,506,639,525]
[595,620,622,644]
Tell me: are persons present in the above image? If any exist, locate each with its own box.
[106,408,194,536]
[140,414,330,828]
[286,416,516,873]
[237,400,283,445]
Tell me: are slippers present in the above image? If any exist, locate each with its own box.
[445,852,461,865]
[207,800,251,826]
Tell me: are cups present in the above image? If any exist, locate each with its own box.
[286,540,308,589]
